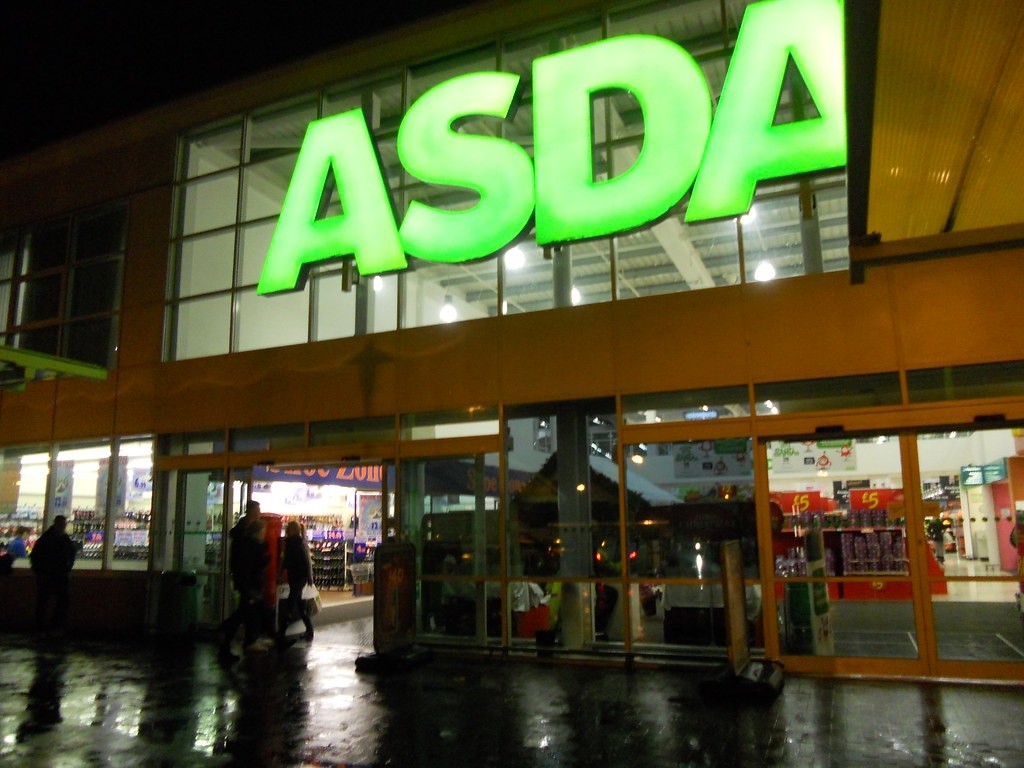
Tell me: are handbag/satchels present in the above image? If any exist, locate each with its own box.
[301,580,323,619]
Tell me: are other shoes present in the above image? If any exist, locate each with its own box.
[278,634,296,649]
[217,650,240,661]
[304,623,314,639]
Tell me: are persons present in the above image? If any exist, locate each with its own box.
[29,514,82,630]
[7,526,30,560]
[279,521,313,641]
[216,501,297,660]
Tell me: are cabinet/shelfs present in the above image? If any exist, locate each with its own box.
[0,509,351,592]
[782,526,908,577]
